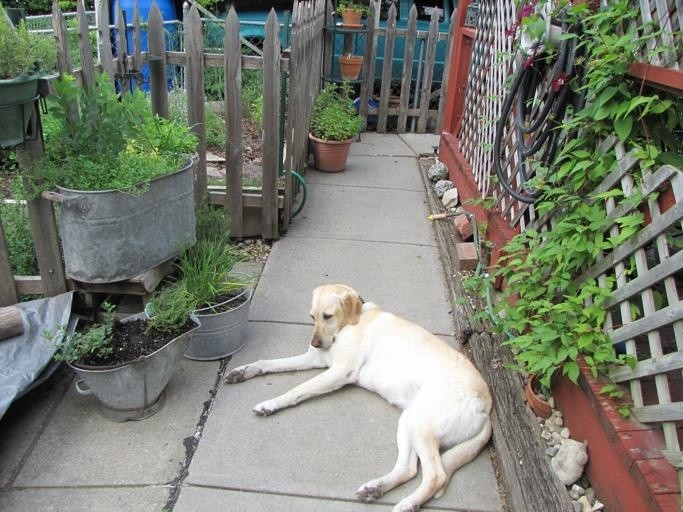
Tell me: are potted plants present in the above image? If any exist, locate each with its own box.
[1,11,58,107]
[43,287,202,423]
[308,1,373,173]
[8,70,199,284]
[144,211,254,362]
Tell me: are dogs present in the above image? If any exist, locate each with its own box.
[223,283,494,512]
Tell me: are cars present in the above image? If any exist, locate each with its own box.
[203,0,453,85]
